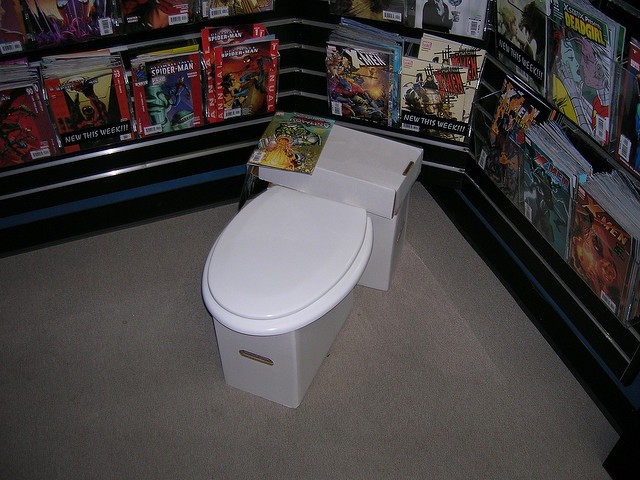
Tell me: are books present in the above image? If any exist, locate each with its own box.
[1,1,204,168]
[247,110,337,178]
[477,1,639,323]
[203,1,281,124]
[326,1,491,153]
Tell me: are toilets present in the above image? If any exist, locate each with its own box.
[200,112,426,408]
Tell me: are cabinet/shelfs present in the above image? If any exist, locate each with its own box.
[1,0,638,480]
